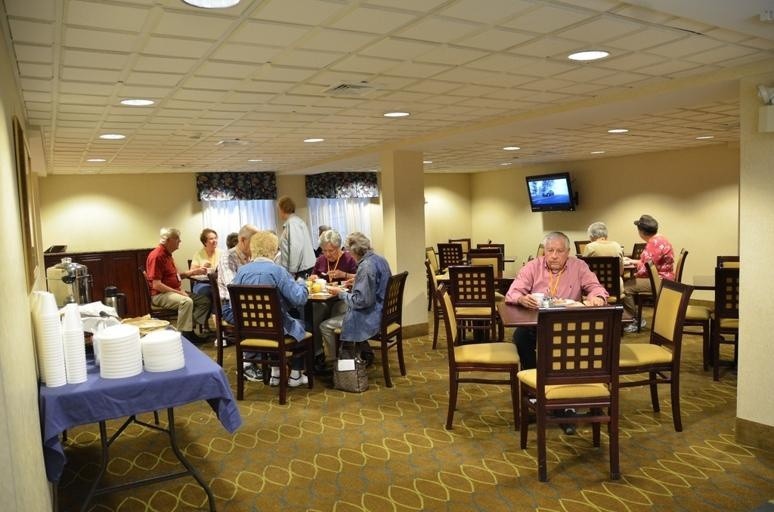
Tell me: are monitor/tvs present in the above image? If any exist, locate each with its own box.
[526,173,576,212]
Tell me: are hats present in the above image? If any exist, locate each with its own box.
[634,215,658,233]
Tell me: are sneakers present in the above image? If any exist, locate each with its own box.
[622,319,647,334]
[214,334,227,347]
[269,375,280,386]
[286,374,308,386]
[236,365,264,382]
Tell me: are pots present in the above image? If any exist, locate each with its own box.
[47,256,94,305]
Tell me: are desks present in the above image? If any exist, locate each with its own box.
[39,318,242,512]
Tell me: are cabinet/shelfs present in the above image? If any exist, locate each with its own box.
[44,248,156,319]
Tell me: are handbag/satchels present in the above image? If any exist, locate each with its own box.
[332,355,369,393]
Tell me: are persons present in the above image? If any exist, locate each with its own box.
[505,232,610,434]
[623,215,675,332]
[278,198,316,280]
[583,222,625,295]
[148,227,210,345]
[190,228,224,332]
[217,224,390,387]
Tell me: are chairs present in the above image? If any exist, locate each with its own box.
[139,264,178,321]
[207,273,234,367]
[188,260,215,334]
[227,284,313,405]
[334,271,409,387]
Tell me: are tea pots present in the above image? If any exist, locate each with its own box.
[104,286,129,317]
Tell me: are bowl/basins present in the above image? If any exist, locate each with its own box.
[121,318,170,332]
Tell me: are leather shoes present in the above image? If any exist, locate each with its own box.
[182,331,207,344]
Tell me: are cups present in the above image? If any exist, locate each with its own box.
[31,289,89,387]
[207,268,214,274]
[532,292,543,308]
[306,279,326,293]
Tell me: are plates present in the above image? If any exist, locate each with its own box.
[543,298,575,306]
[97,324,142,380]
[142,329,186,373]
